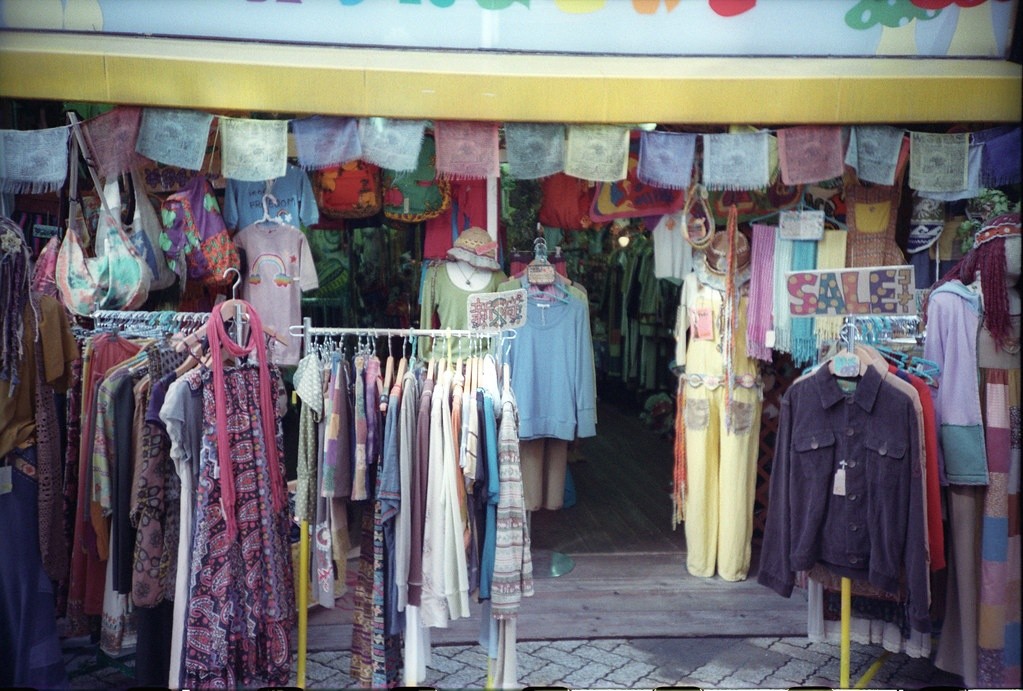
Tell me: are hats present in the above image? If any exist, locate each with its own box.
[973,212,1022,248]
[448,226,501,272]
[692,231,753,290]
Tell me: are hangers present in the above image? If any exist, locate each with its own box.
[779,312,942,407]
[236,193,304,239]
[519,236,590,309]
[88,267,512,413]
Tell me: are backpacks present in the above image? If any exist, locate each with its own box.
[161,175,240,286]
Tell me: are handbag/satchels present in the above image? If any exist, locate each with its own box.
[95,132,176,291]
[54,111,153,317]
